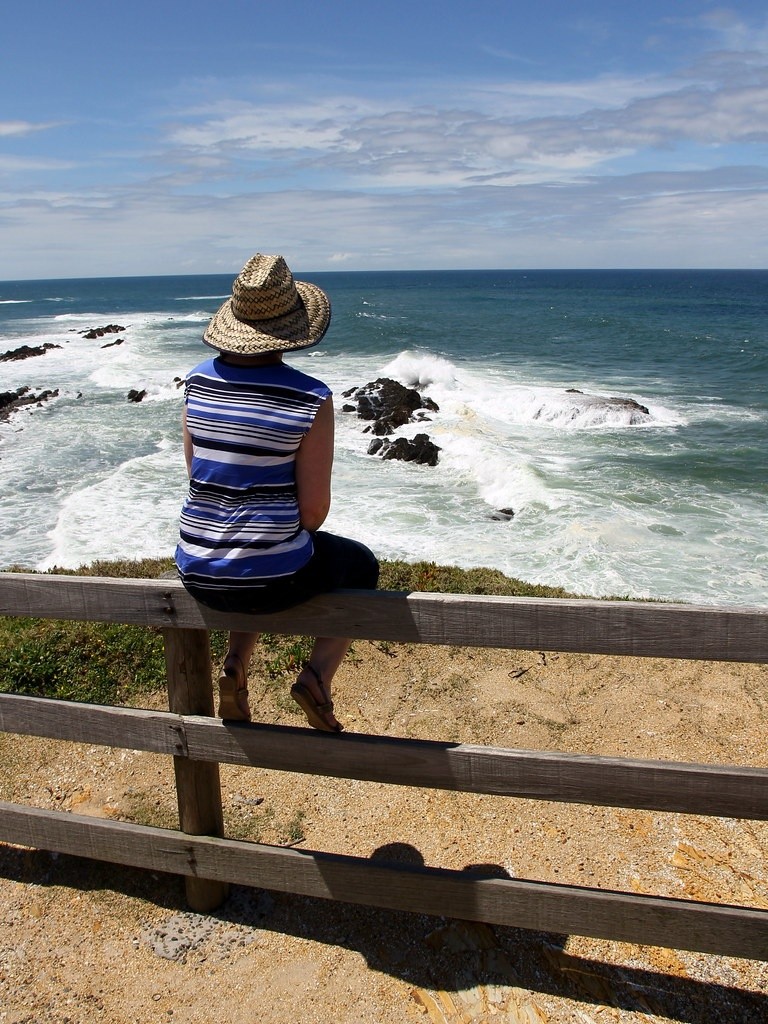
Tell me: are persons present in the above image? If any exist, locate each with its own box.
[174,253,380,733]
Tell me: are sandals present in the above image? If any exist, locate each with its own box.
[217,655,252,722]
[290,662,343,733]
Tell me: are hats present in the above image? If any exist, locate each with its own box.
[202,252,331,356]
